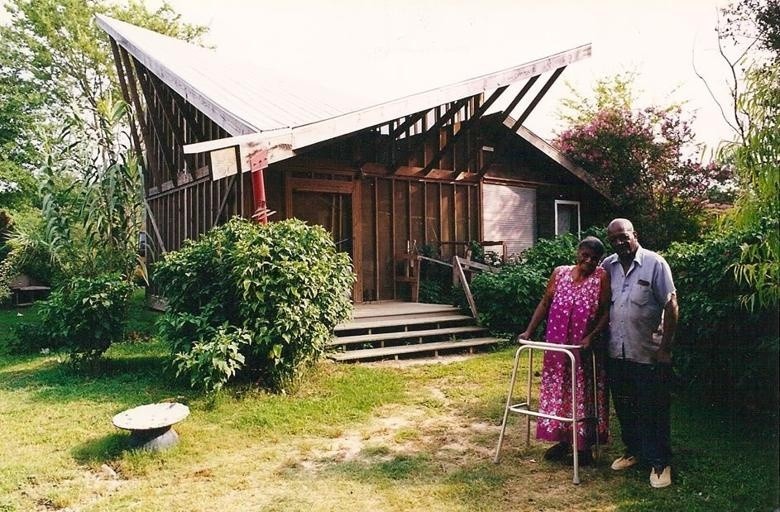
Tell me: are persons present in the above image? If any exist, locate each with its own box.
[597,218,681,489]
[517,235,611,468]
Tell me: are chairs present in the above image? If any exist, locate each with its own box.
[565,450,592,465]
[544,442,571,459]
[612,454,640,471]
[650,466,671,488]
